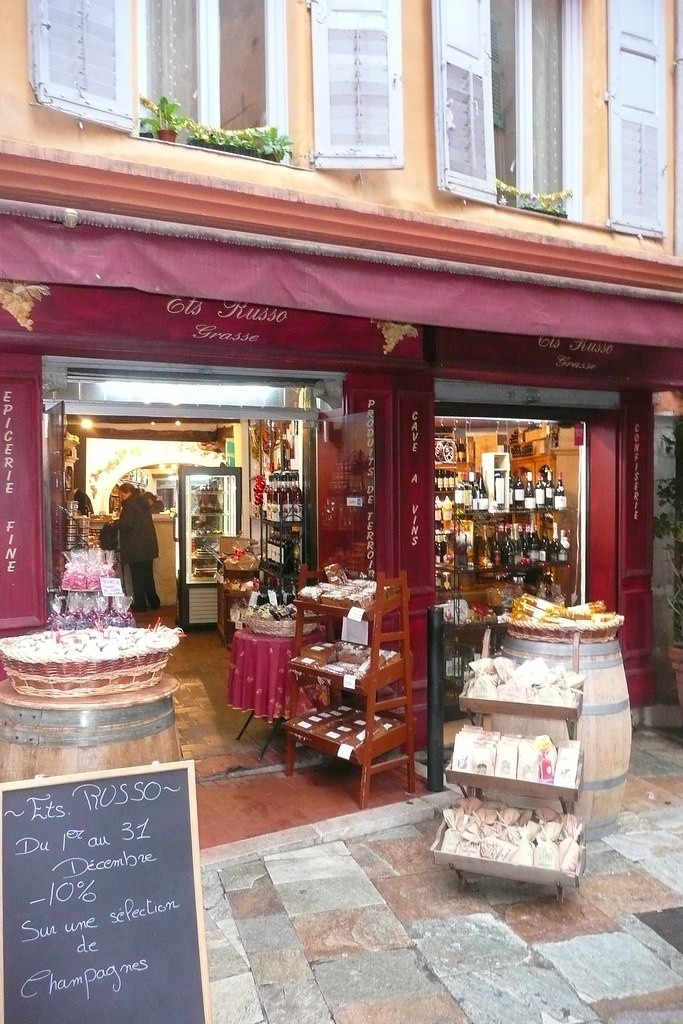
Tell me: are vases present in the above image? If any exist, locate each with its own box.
[522,207,568,219]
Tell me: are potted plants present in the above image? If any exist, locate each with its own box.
[141,95,294,163]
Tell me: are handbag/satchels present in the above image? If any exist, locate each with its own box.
[98,523,118,551]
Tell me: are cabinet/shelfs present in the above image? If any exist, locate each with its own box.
[436,418,591,723]
[285,566,418,811]
[430,628,584,904]
[216,559,259,648]
[258,506,304,605]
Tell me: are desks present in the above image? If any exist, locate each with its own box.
[226,625,330,763]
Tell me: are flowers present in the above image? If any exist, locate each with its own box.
[498,178,572,212]
[653,418,683,709]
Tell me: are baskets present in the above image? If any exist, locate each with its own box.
[242,614,317,637]
[0,628,178,698]
[227,587,257,597]
[505,614,625,643]
[223,556,261,570]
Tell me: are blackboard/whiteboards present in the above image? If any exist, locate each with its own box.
[1,758,211,1024]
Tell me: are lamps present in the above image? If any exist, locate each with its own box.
[652,390,677,416]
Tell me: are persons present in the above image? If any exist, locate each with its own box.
[144,492,164,514]
[74,488,94,518]
[108,483,160,611]
